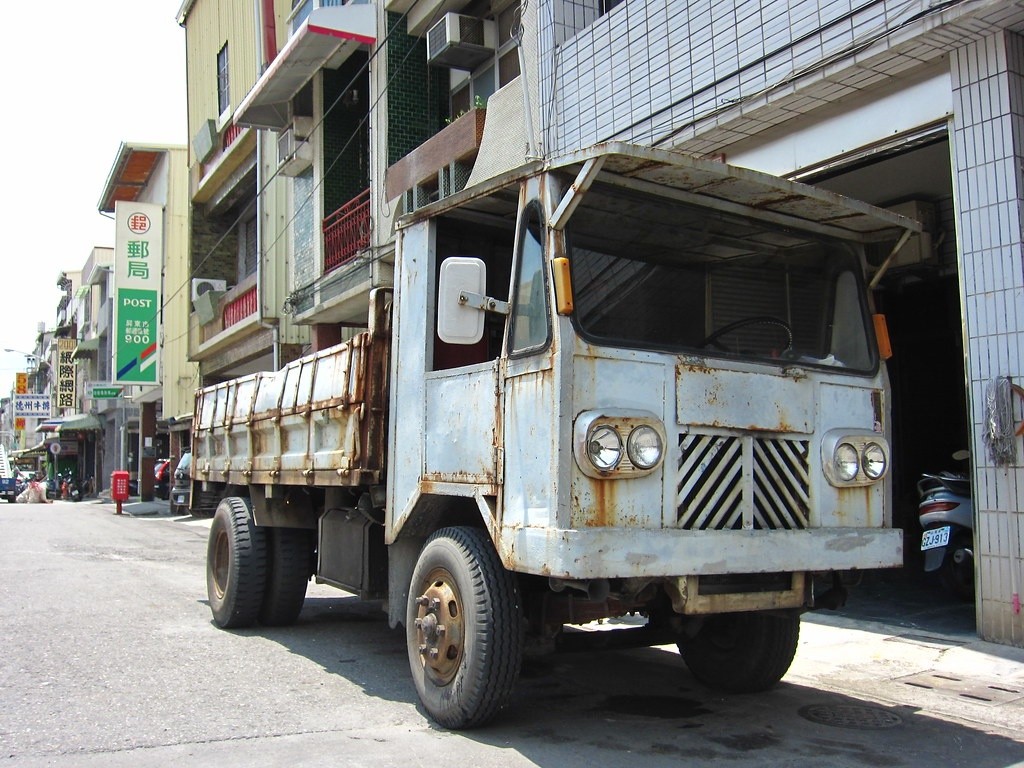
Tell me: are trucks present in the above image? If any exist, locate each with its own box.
[189,141,928,730]
[0,477,18,504]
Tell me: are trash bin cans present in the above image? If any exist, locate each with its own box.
[111,471,129,501]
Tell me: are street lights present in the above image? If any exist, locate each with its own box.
[4,348,57,386]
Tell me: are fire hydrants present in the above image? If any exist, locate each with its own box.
[61,481,69,499]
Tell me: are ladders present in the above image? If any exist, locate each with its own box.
[0,444,7,478]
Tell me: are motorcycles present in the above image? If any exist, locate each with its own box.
[914,470,977,600]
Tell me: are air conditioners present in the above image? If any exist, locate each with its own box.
[426,12,496,71]
[857,199,938,276]
[192,278,227,302]
[275,129,294,169]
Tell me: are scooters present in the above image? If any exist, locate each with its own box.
[58,470,89,502]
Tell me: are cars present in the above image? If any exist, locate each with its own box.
[153,457,170,499]
[170,451,223,516]
